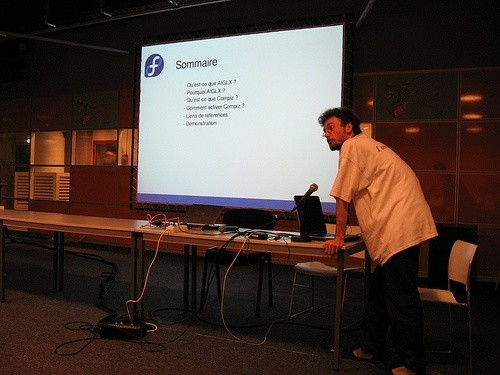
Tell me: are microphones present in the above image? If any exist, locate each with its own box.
[155,221,178,226]
[219,226,241,233]
[290,183,318,214]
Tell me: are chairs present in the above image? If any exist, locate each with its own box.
[289,223,372,331]
[199,208,276,317]
[418,239,480,365]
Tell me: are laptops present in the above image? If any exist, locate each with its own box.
[294,195,360,241]
[183,203,227,227]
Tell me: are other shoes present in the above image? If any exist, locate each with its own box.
[353,349,372,361]
[392,365,415,375]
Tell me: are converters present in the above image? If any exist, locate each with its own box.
[246,231,269,239]
[102,323,147,340]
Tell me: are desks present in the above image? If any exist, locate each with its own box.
[0,210,319,323]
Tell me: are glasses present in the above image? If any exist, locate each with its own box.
[322,124,348,137]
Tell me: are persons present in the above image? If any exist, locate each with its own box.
[318,106,438,375]
